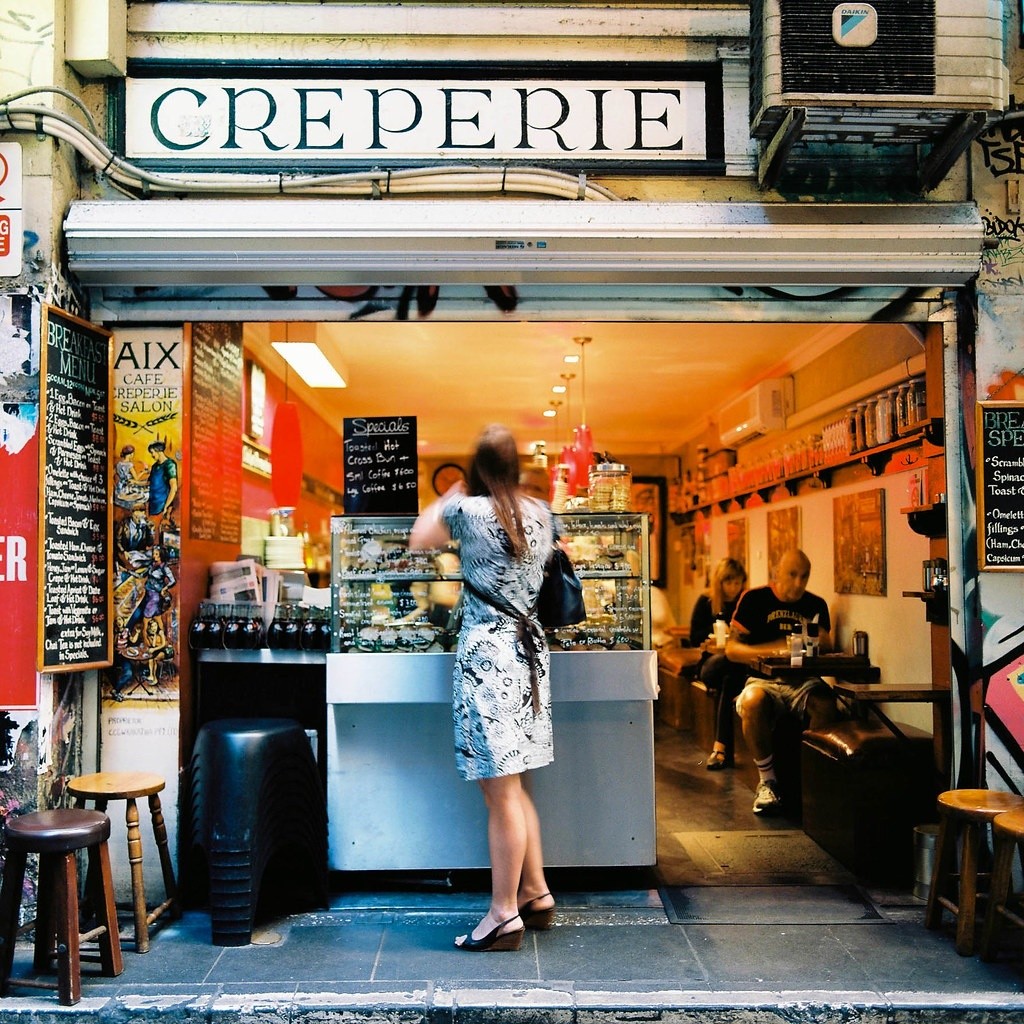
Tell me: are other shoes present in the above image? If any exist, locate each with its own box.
[752,778,783,815]
[706,750,727,769]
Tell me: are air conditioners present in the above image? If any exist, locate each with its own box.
[749,0,1014,157]
[716,375,795,453]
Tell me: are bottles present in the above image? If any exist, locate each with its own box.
[787,622,820,668]
[726,377,927,494]
[853,631,869,658]
[715,612,728,649]
[921,557,949,592]
[588,462,634,513]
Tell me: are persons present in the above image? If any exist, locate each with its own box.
[688,557,750,770]
[651,587,681,655]
[517,461,551,506]
[726,548,838,818]
[409,426,572,953]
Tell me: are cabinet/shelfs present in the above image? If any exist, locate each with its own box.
[899,416,949,601]
[325,510,653,652]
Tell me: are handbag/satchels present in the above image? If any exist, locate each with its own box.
[534,547,589,628]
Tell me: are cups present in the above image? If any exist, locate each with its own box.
[535,441,569,513]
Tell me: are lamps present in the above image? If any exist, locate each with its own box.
[270,322,351,389]
[270,322,304,514]
[548,399,565,500]
[570,335,597,489]
[558,372,578,494]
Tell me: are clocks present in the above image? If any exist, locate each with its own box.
[431,462,468,496]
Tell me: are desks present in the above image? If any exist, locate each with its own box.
[698,638,728,656]
[661,625,691,640]
[829,681,936,766]
[747,651,879,717]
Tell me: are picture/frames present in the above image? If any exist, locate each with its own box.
[630,475,671,588]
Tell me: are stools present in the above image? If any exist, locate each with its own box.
[65,769,181,952]
[660,647,704,731]
[795,715,934,871]
[923,787,1024,957]
[688,678,727,757]
[0,810,124,1008]
[729,692,844,821]
[981,809,1024,981]
[188,716,331,950]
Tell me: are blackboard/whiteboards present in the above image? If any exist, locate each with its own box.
[344,416,419,517]
[975,400,1024,572]
[35,300,112,673]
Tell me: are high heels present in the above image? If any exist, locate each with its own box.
[518,893,556,931]
[454,914,525,951]
